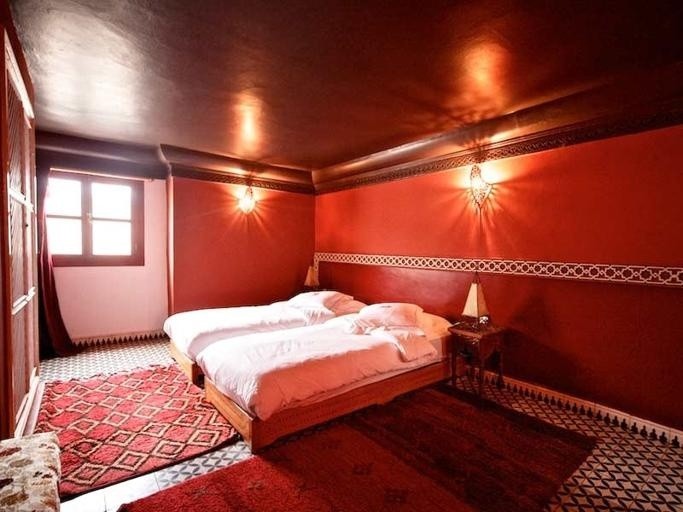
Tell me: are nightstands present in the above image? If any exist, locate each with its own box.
[448,320,505,399]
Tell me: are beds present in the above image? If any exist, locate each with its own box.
[163,300,367,385]
[195,312,467,455]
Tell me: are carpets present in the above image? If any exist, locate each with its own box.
[31,359,237,497]
[116,380,595,512]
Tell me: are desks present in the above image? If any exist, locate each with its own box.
[0,430,62,512]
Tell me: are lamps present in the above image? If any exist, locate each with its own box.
[460,267,490,329]
[468,160,493,209]
[303,265,320,291]
[237,186,256,214]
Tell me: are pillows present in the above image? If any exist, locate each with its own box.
[288,291,354,312]
[358,301,424,326]
